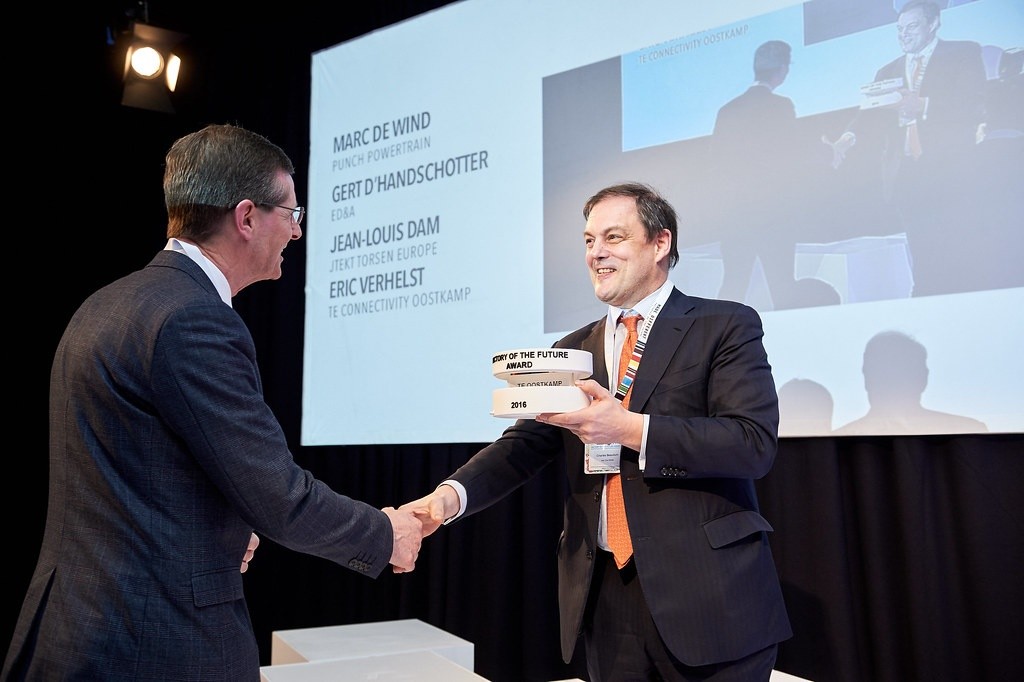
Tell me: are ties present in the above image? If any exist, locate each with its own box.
[909,55,925,155]
[607,314,644,570]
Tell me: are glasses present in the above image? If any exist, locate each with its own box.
[263,203,305,225]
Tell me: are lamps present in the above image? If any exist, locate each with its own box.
[119,0,181,111]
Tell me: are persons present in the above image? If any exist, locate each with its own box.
[773,332,989,436]
[0,124,422,682]
[397,186,794,682]
[834,1,1007,296]
[711,42,845,308]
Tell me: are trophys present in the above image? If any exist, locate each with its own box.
[492,348,593,420]
[859,78,903,110]
